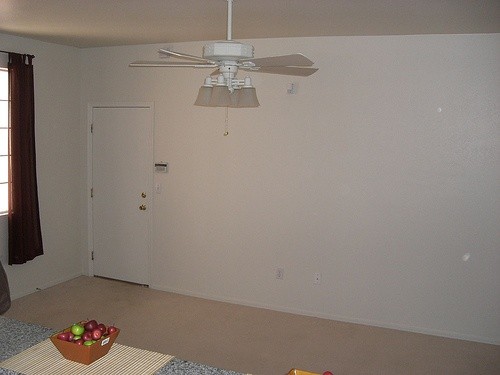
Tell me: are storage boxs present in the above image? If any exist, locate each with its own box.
[50,320,120,365]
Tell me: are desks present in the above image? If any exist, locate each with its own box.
[0,317,250,375]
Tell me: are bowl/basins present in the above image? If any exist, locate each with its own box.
[50,320,120,364]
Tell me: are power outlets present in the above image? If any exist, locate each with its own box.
[276,270,284,279]
[314,273,320,282]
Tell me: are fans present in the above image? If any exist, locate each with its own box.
[128,0,319,77]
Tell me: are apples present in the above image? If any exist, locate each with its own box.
[57,319,118,345]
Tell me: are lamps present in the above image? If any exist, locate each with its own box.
[193,86,260,135]
